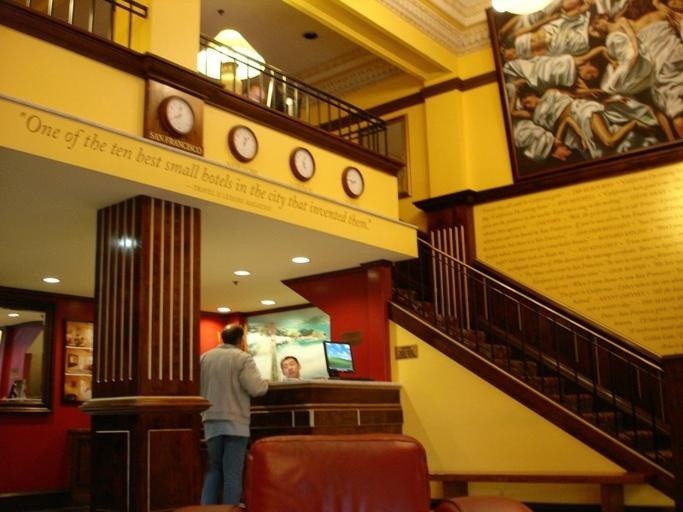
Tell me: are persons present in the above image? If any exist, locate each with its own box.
[280,356,301,378]
[197,324,270,504]
[490,1,682,167]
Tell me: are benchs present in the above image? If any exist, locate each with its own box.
[427,473,656,511]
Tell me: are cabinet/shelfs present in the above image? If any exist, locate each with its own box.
[62,319,94,404]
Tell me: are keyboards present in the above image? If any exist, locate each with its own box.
[329,377,375,380]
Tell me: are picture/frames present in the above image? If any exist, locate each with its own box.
[484,1,683,183]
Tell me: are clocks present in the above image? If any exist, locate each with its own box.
[290,143,316,183]
[158,92,197,140]
[342,165,366,200]
[228,125,259,164]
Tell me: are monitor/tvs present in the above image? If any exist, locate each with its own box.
[322,341,357,379]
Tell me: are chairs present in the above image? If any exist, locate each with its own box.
[176,434,532,512]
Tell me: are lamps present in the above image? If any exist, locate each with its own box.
[198,27,266,94]
